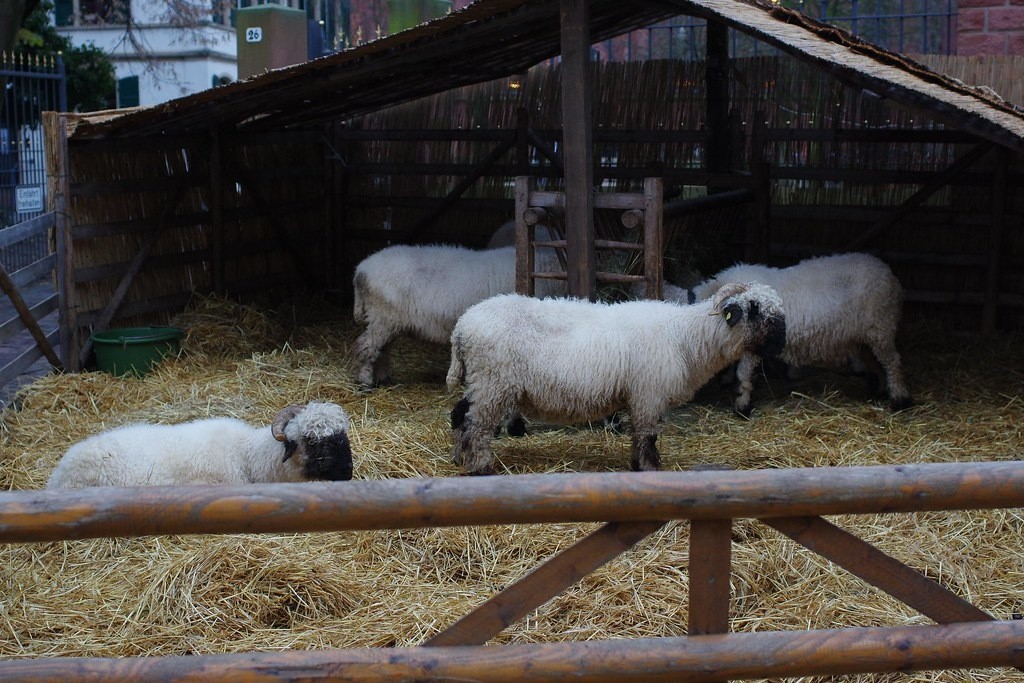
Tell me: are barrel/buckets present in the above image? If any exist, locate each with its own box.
[91,326,182,375]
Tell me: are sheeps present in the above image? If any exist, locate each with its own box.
[446,279,788,476]
[45,400,353,494]
[353,220,568,388]
[629,253,909,420]
